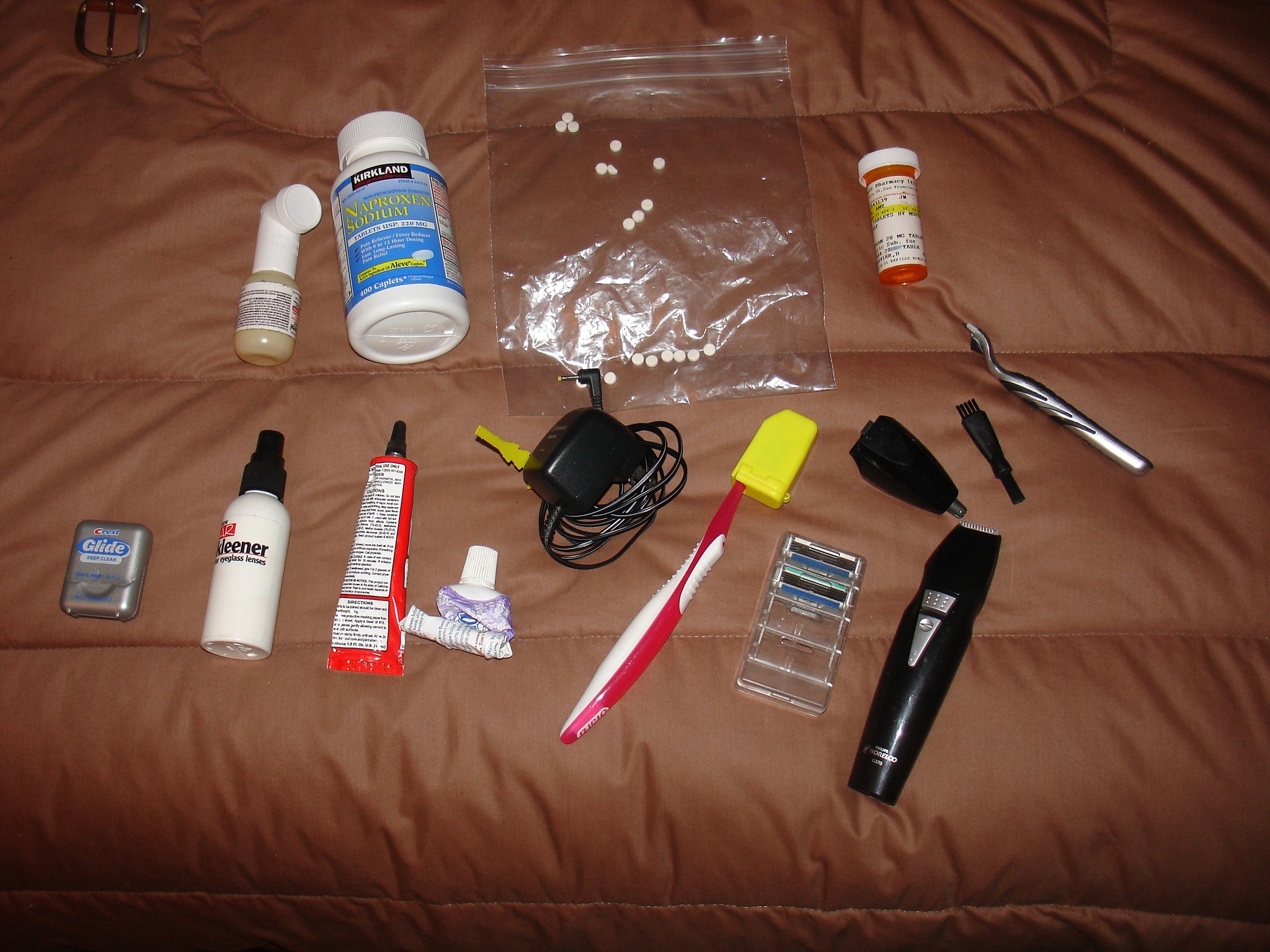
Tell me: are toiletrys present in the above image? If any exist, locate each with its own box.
[401,543,518,659]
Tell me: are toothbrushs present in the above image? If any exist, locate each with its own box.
[555,479,746,747]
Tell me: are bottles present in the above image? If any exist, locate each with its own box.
[200,430,291,659]
[233,183,322,368]
[329,112,470,364]
[857,147,928,287]
